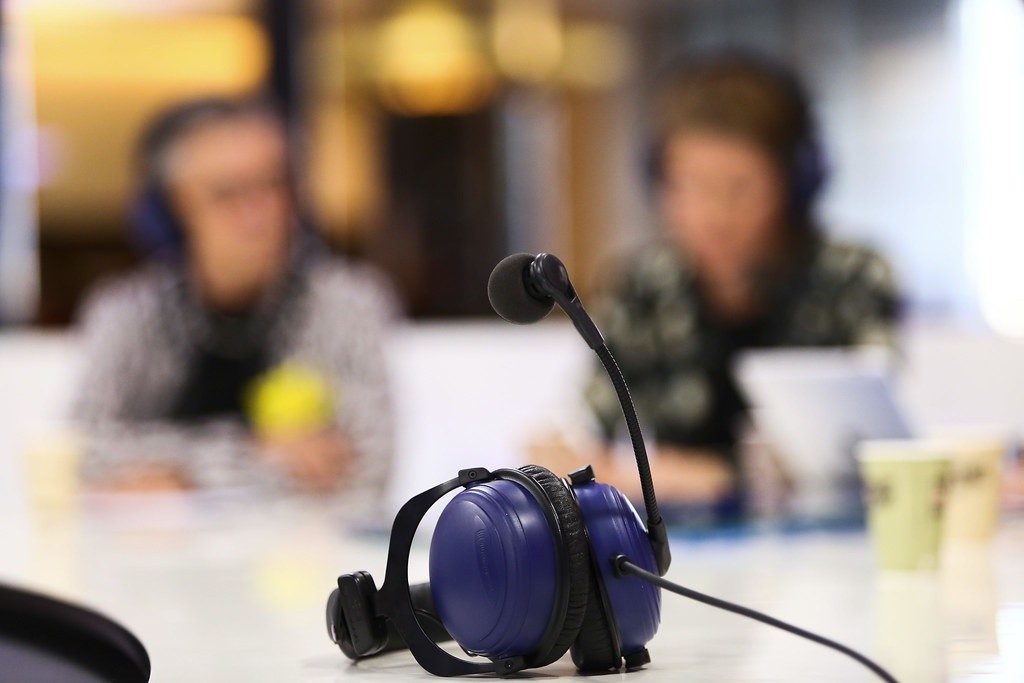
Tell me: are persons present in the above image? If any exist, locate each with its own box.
[513,46,928,522]
[38,97,414,499]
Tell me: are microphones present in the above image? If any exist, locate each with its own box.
[490,251,672,577]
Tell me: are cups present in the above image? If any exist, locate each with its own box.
[930,423,1002,543]
[855,441,947,567]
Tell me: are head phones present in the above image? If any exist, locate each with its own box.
[325,463,663,679]
[133,99,243,266]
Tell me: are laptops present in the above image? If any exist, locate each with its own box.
[738,345,920,524]
